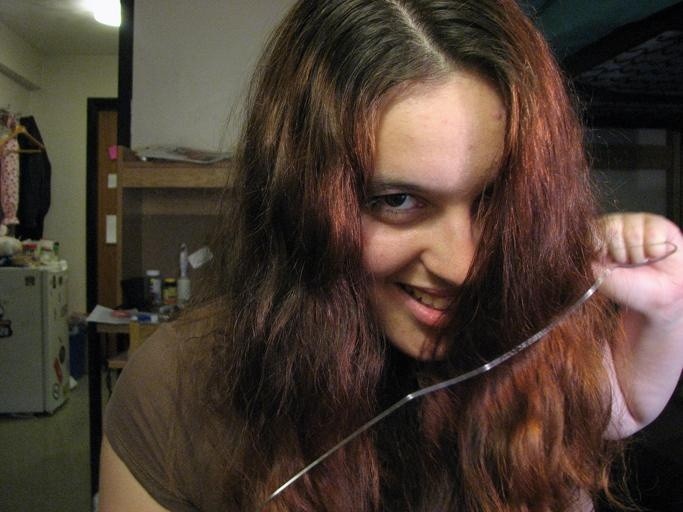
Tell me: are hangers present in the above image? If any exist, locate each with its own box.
[0,115,47,155]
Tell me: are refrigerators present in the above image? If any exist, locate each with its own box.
[0,257,70,415]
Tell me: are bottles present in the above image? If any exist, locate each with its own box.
[146,269,190,309]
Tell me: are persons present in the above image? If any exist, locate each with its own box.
[98,0,682,511]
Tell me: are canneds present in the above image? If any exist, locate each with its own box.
[146,269,162,306]
[161,278,176,306]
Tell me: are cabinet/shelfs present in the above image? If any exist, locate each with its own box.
[105,143,237,396]
